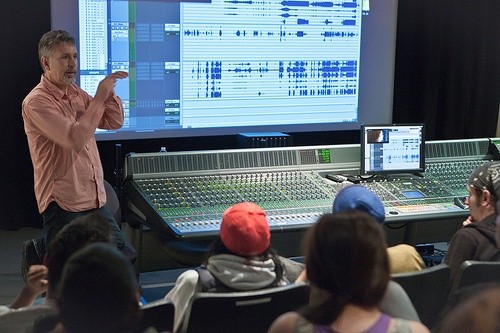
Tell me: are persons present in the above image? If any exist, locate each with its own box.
[165,204,305,333]
[295,185,427,283]
[441,161,500,269]
[435,283,500,333]
[22,30,128,255]
[270,214,431,333]
[2,215,149,333]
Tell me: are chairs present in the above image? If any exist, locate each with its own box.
[1,260,500,333]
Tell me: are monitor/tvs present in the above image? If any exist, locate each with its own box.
[360,124,425,173]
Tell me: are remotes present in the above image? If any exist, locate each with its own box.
[327,174,360,184]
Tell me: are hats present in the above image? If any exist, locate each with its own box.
[333,185,385,223]
[467,162,500,200]
[58,243,137,333]
[220,202,271,256]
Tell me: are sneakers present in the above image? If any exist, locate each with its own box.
[21,240,39,283]
